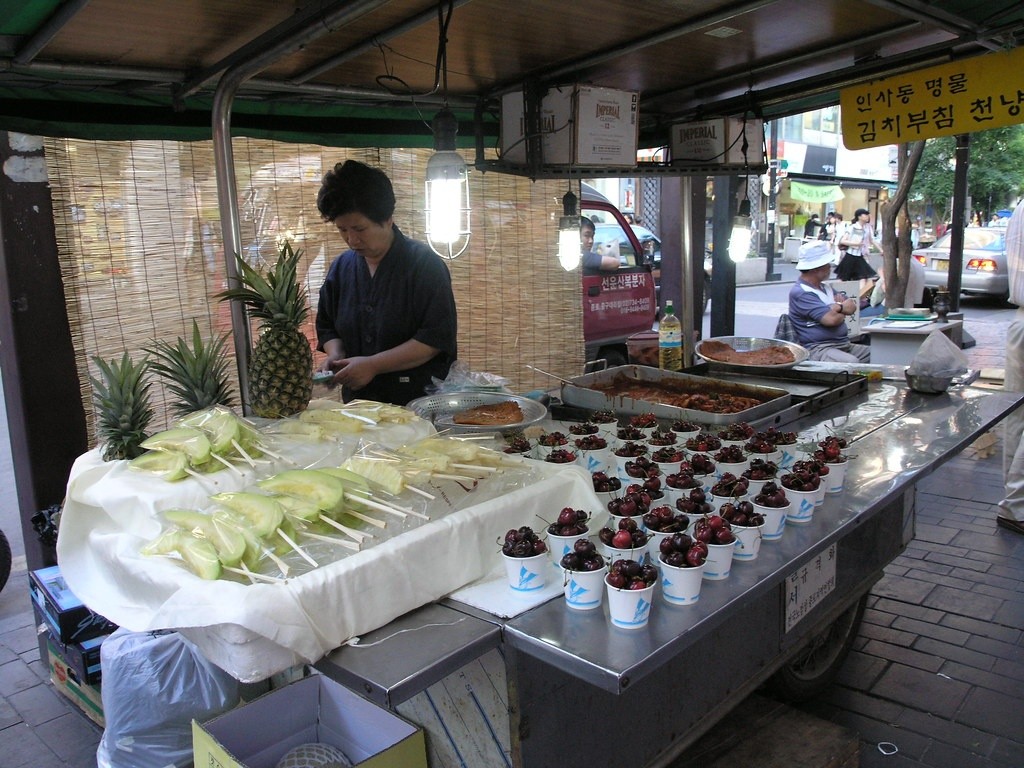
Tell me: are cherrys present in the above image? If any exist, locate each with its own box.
[498,408,859,590]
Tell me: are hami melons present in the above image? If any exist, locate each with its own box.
[130,408,371,583]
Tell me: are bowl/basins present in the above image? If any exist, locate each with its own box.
[905,370,954,394]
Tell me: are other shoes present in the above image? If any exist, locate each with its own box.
[996,515,1024,534]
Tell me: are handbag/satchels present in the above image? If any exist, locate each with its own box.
[838,236,849,250]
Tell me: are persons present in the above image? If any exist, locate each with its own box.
[967,219,980,228]
[787,238,871,365]
[802,213,823,245]
[623,214,633,225]
[832,209,883,289]
[987,213,1000,227]
[580,215,621,271]
[635,215,651,231]
[314,159,459,406]
[996,200,1024,533]
[818,211,849,266]
[858,237,925,318]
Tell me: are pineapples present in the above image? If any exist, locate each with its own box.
[215,240,315,418]
[278,401,492,496]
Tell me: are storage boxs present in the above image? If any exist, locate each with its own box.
[498,84,640,169]
[192,674,428,768]
[824,280,859,336]
[670,117,762,166]
[27,565,106,729]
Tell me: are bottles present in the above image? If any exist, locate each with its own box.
[659,300,683,372]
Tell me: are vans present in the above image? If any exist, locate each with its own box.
[581,182,660,369]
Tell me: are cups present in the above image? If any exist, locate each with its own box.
[503,419,848,631]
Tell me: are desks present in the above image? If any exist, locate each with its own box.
[783,236,818,263]
[860,319,962,366]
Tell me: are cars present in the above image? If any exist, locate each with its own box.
[590,222,711,323]
[912,225,1017,309]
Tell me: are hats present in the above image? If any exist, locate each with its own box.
[796,241,834,270]
